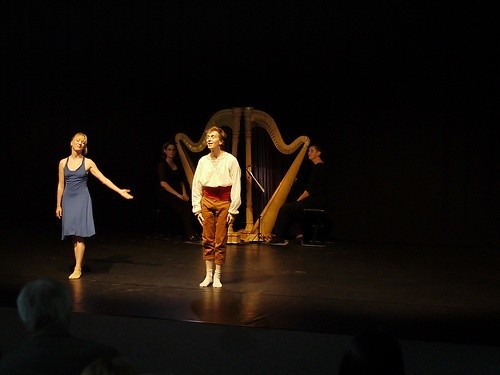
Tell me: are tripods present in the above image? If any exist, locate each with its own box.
[245,168,267,245]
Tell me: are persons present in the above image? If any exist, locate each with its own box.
[268,144,326,246]
[192,126,242,289]
[56,133,134,280]
[156,141,202,244]
